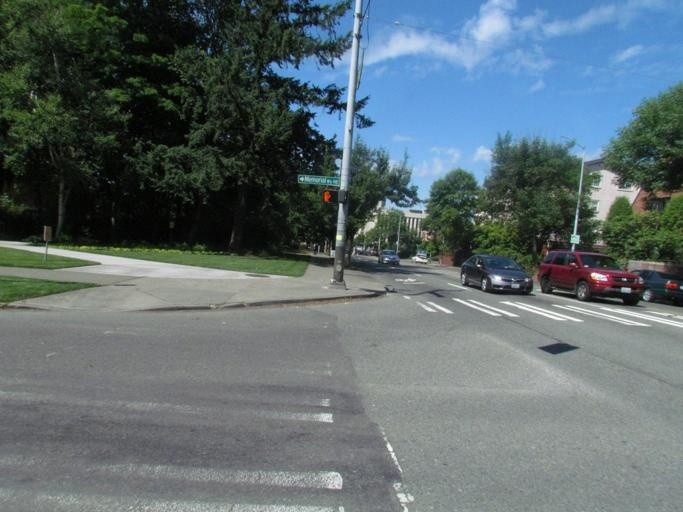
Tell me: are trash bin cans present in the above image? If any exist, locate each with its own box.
[330,251,336,258]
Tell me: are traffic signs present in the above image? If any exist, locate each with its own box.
[298,175,340,186]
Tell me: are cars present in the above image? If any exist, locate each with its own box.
[378,250,400,266]
[630,270,683,305]
[461,255,533,294]
[412,254,428,264]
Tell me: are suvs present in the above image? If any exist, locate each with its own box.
[538,251,644,306]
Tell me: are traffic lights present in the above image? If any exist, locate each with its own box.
[321,189,338,203]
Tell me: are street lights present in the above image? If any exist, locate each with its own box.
[560,135,586,252]
